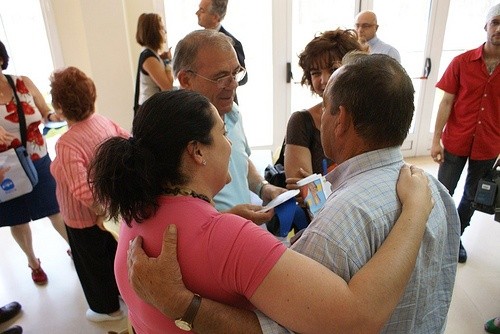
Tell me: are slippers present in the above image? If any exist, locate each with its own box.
[86,308,126,322]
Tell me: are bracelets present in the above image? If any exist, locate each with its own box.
[48,113,52,121]
[165,63,172,72]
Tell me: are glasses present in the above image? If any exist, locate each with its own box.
[188,66,248,88]
[354,23,377,30]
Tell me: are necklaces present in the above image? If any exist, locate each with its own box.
[160,185,216,208]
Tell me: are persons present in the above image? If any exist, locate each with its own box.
[355,11,401,65]
[484,316,500,334]
[87,89,435,334]
[127,54,461,334]
[430,1,500,264]
[87,88,437,334]
[133,13,178,116]
[175,29,289,225]
[50,65,133,322]
[263,26,374,247]
[195,0,247,104]
[0,40,73,285]
[0,301,23,334]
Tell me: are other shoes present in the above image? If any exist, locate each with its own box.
[0,324,24,334]
[28,258,47,285]
[0,301,24,329]
[458,239,468,263]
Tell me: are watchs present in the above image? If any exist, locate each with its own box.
[175,292,202,332]
[256,180,268,198]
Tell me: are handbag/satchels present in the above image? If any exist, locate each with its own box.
[259,108,317,239]
[0,143,39,205]
[472,156,500,215]
